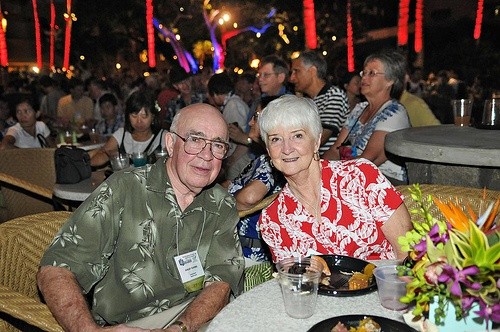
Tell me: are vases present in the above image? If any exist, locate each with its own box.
[428,294,493,332]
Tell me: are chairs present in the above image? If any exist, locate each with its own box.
[0,145,72,213]
[0,210,72,332]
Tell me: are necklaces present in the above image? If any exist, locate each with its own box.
[289,172,320,221]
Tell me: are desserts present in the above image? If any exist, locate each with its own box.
[348,264,376,289]
[331,317,382,332]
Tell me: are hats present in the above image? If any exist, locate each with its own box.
[166,65,193,87]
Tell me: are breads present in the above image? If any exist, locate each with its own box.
[310,256,331,276]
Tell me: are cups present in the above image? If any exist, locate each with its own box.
[452,99,472,127]
[372,264,418,311]
[481,97,500,129]
[131,151,147,167]
[276,256,324,319]
[155,147,167,159]
[110,152,130,172]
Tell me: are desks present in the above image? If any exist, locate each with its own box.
[49,131,109,152]
[383,120,500,192]
[205,259,500,332]
[53,170,105,202]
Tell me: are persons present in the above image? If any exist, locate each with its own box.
[256,95,413,272]
[0,51,500,268]
[36,105,245,332]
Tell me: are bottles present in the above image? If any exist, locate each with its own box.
[92,128,96,144]
[65,131,72,144]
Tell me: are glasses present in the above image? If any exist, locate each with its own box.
[256,72,280,79]
[172,131,232,160]
[359,70,386,78]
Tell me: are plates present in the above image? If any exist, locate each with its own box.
[306,314,419,332]
[288,254,386,297]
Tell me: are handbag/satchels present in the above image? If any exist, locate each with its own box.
[54,145,92,184]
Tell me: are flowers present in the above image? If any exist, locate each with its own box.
[396,183,500,326]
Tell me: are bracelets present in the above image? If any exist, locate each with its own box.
[171,321,187,332]
